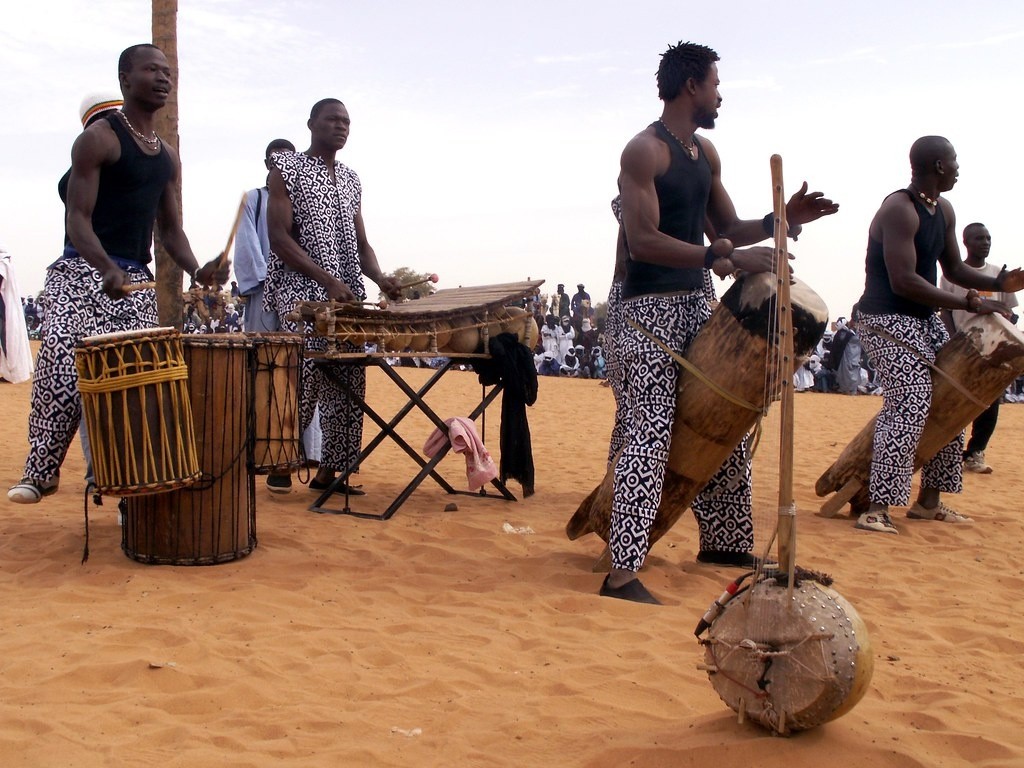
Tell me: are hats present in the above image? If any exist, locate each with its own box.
[80,93,125,131]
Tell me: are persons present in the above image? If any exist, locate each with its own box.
[0,249,35,385]
[234,139,322,468]
[260,97,405,496]
[57,89,124,484]
[8,44,232,506]
[20,279,1024,404]
[939,222,1019,473]
[598,40,839,607]
[856,135,1024,534]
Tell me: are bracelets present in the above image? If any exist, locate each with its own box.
[763,213,802,242]
[704,236,735,281]
[195,267,201,280]
[965,288,982,312]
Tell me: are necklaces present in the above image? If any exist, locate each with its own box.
[659,117,695,157]
[913,185,938,207]
[117,110,160,151]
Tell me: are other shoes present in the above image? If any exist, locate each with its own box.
[308,478,365,496]
[266,473,292,493]
[599,573,661,606]
[698,548,779,570]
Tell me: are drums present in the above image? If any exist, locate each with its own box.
[121,334,258,565]
[73,327,203,499]
[239,332,304,474]
[815,312,1024,518]
[566,270,829,573]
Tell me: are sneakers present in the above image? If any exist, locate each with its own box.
[7,476,59,504]
[962,450,993,474]
[854,511,899,534]
[906,502,974,524]
